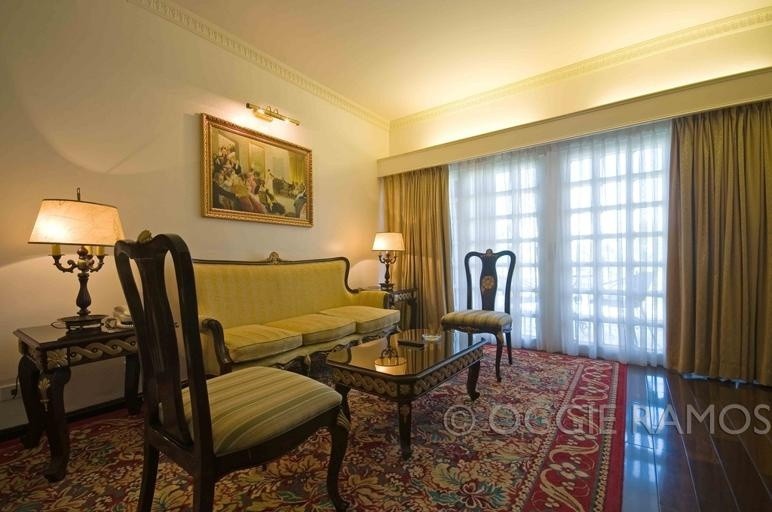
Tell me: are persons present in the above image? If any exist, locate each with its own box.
[213,144,307,219]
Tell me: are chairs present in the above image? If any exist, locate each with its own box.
[440,248,516,383]
[114,228,355,512]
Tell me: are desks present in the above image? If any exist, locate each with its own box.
[359,288,418,330]
[12,315,180,483]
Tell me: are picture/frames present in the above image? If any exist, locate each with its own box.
[200,112,314,228]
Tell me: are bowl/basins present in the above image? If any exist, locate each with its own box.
[423,333,442,342]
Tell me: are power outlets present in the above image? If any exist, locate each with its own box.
[0,383,22,402]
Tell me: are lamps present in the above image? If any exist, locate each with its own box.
[27,188,126,329]
[374,331,407,375]
[370,232,406,292]
[246,103,301,127]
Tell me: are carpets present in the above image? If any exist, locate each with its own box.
[0,343,628,512]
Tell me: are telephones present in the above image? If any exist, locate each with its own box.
[113,306,132,323]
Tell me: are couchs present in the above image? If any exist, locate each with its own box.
[170,251,400,379]
